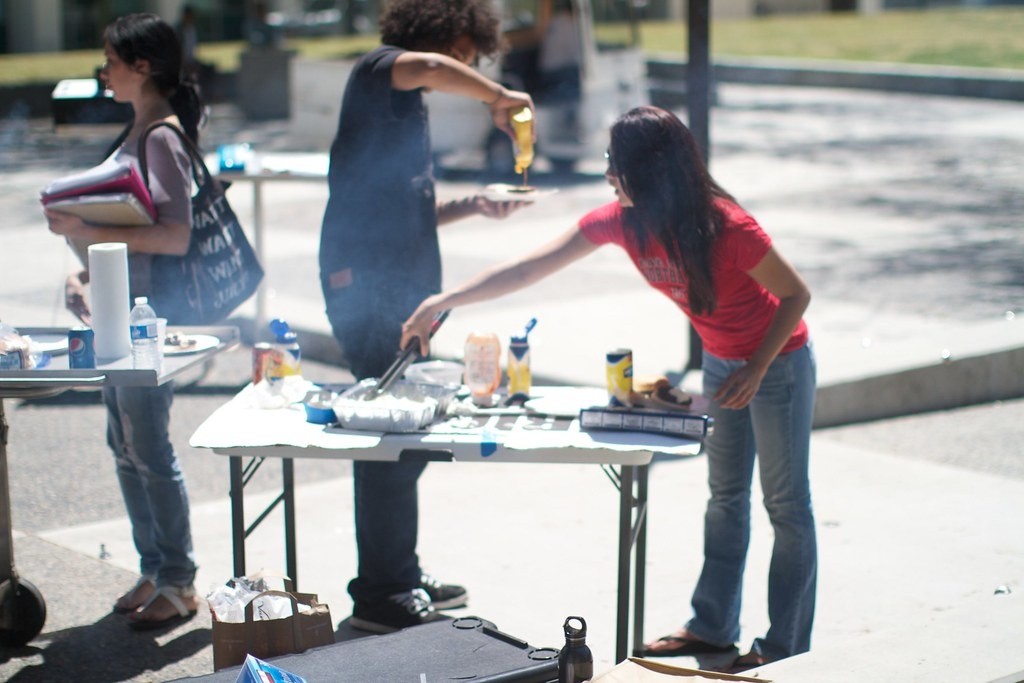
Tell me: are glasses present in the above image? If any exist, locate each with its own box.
[604,146,626,177]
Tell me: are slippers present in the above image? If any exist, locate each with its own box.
[635,631,736,656]
[709,653,774,674]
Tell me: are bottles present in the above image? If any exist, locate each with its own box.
[558,617,593,683]
[129,296,160,370]
[506,335,532,400]
[509,104,535,174]
[463,330,502,408]
[267,322,303,386]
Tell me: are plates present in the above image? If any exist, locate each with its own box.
[484,183,556,203]
[523,391,612,418]
[161,335,221,356]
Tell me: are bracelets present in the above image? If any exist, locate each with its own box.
[481,85,503,105]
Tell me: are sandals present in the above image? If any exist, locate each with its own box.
[116,575,201,626]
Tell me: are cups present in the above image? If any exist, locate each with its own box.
[156,317,167,346]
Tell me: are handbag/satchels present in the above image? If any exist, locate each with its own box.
[141,120,266,325]
[210,589,336,672]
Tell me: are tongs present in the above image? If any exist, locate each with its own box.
[363,307,458,403]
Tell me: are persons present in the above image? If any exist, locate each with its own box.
[318,1,535,630]
[396,105,817,672]
[483,1,554,180]
[47,14,199,627]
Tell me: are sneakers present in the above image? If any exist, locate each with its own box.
[350,572,468,634]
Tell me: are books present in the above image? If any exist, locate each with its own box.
[38,159,158,275]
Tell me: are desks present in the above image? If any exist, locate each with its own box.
[190,376,701,667]
[191,147,332,354]
[0,326,241,650]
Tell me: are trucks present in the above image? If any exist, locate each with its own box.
[289,0,654,180]
